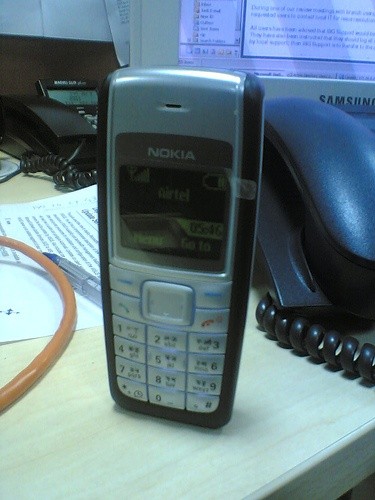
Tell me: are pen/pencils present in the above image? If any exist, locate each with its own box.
[43,252,103,306]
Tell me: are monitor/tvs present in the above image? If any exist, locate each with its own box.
[128,0,375,106]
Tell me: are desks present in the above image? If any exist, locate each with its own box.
[1,152,375,500]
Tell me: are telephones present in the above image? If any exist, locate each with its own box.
[0,78,101,190]
[256,98,375,384]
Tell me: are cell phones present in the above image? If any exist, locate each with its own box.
[98,65,265,429]
